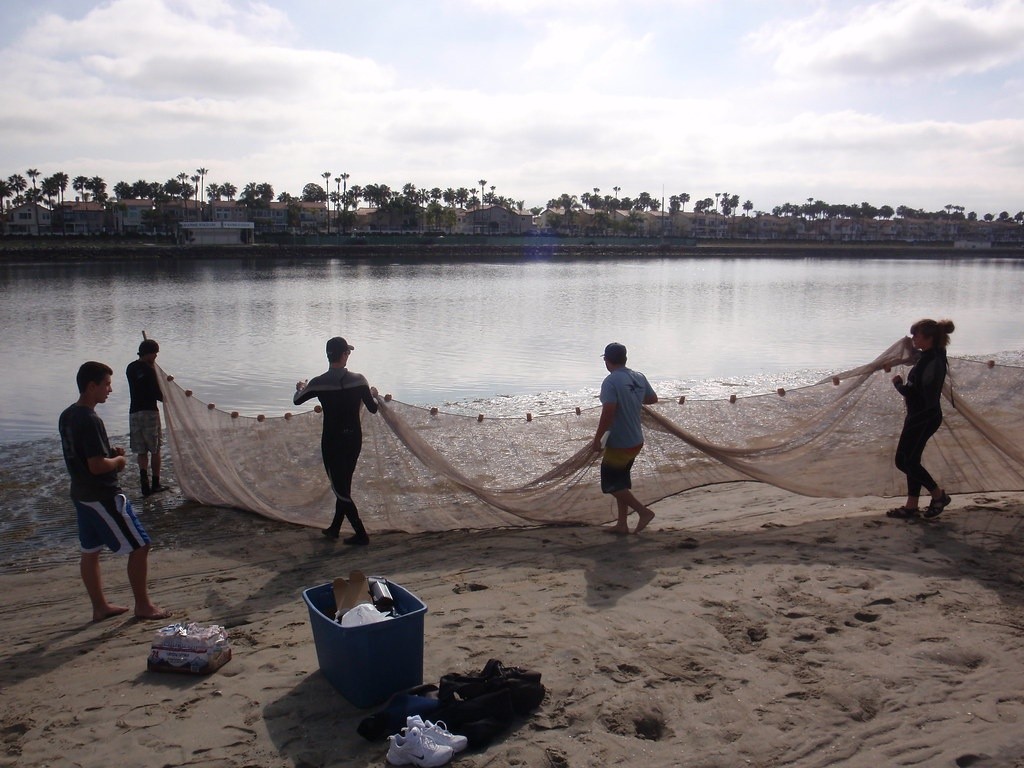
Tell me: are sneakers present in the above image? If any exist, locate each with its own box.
[386,727,454,767]
[401,715,468,752]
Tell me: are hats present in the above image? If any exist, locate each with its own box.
[601,343,626,359]
[137,340,158,355]
[326,337,355,356]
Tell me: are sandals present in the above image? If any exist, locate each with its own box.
[886,505,920,519]
[924,489,952,518]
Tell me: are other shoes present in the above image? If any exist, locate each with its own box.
[140,476,153,496]
[343,531,369,545]
[154,483,170,491]
[323,526,339,538]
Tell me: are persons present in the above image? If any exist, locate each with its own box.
[126,339,170,494]
[293,336,379,544]
[886,319,955,519]
[592,343,658,533]
[58,361,173,622]
